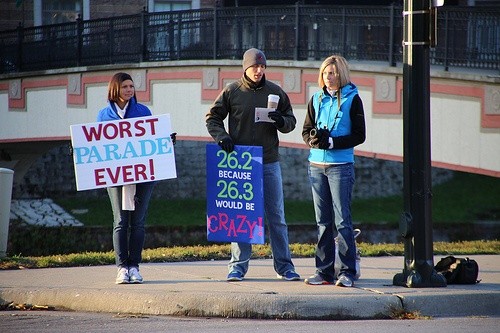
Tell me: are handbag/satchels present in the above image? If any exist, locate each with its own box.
[437,255,481,285]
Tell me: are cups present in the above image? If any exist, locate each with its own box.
[267,94,280,110]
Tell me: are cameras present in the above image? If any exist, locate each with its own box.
[311,128,327,146]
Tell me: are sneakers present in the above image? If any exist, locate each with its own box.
[303,274,333,284]
[275,270,301,280]
[115,267,129,283]
[336,276,353,287]
[129,268,144,283]
[226,272,245,281]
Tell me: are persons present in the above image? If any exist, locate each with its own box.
[302,55,366,287]
[206,47,301,280]
[69,72,177,283]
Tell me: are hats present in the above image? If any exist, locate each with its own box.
[242,48,267,70]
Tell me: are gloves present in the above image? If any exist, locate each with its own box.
[267,110,284,128]
[220,139,234,153]
[309,137,334,150]
[68,145,73,156]
[170,132,177,145]
